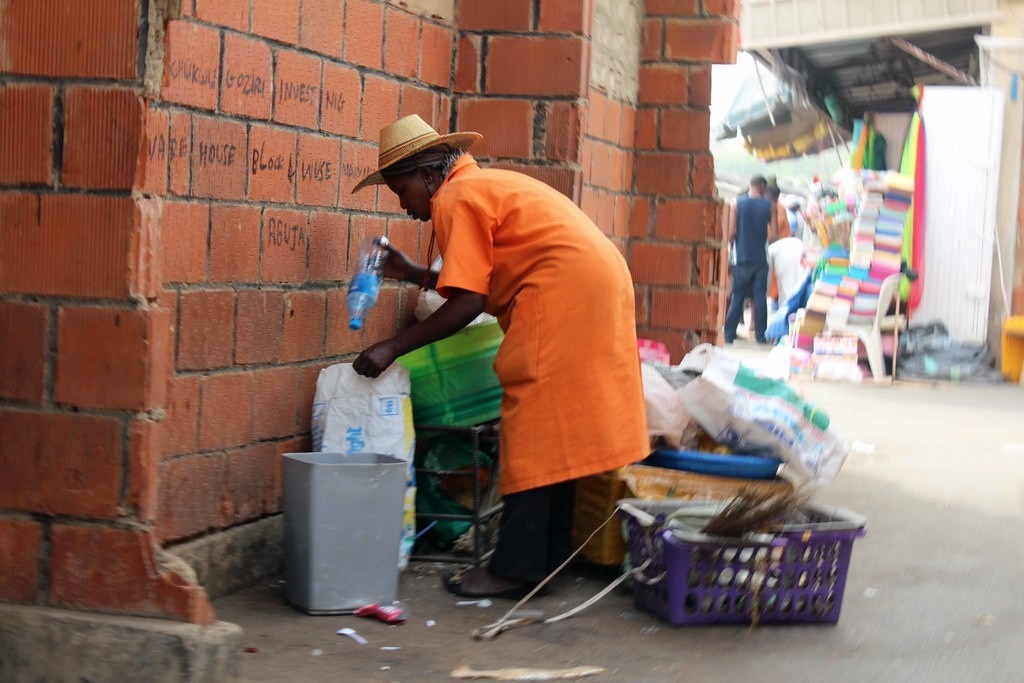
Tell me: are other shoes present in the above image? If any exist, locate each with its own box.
[725,337,734,343]
[441,569,525,601]
[757,337,766,343]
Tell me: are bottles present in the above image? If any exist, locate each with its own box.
[346,235,389,330]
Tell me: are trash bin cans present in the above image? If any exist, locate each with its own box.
[282,452,409,616]
[1002,314,1024,383]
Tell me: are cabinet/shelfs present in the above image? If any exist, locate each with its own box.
[907,84,1005,345]
[407,418,505,569]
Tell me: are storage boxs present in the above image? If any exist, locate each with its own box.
[396,318,505,439]
[572,462,794,565]
[276,445,420,616]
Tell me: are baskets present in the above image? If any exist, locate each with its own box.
[617,497,867,625]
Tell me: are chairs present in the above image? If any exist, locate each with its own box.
[802,265,904,379]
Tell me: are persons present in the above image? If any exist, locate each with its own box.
[350,114,651,595]
[724,175,794,345]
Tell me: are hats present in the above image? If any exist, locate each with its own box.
[350,113,483,195]
